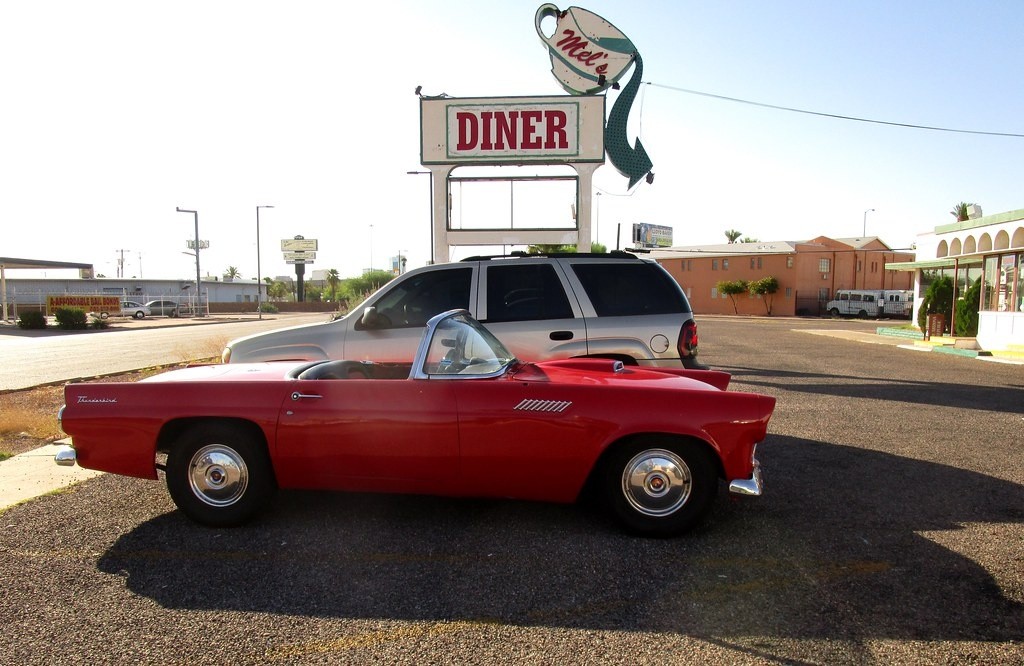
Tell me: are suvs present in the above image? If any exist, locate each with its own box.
[218,248,709,378]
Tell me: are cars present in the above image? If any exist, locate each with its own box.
[90,301,152,320]
[177,303,189,314]
[142,300,180,317]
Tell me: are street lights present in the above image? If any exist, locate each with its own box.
[864,209,876,238]
[256,204,275,320]
[176,205,203,317]
[407,170,434,263]
[594,191,603,246]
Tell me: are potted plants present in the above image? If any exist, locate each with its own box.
[950,201,969,221]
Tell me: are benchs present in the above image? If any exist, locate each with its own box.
[299,360,372,380]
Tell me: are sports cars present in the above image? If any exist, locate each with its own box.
[57,306,776,534]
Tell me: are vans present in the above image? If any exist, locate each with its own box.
[885,290,915,318]
[825,289,883,317]
